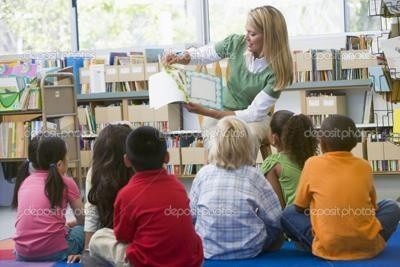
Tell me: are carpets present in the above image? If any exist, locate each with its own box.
[0,222,400,267]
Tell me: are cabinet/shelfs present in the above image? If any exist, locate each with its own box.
[1,70,84,202]
[79,91,205,180]
[284,77,400,176]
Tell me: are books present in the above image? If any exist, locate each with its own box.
[148,53,225,114]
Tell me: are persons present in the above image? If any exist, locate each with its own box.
[86,124,204,267]
[281,112,400,263]
[11,131,85,263]
[188,116,284,262]
[257,110,320,242]
[165,3,295,123]
[64,123,133,264]
